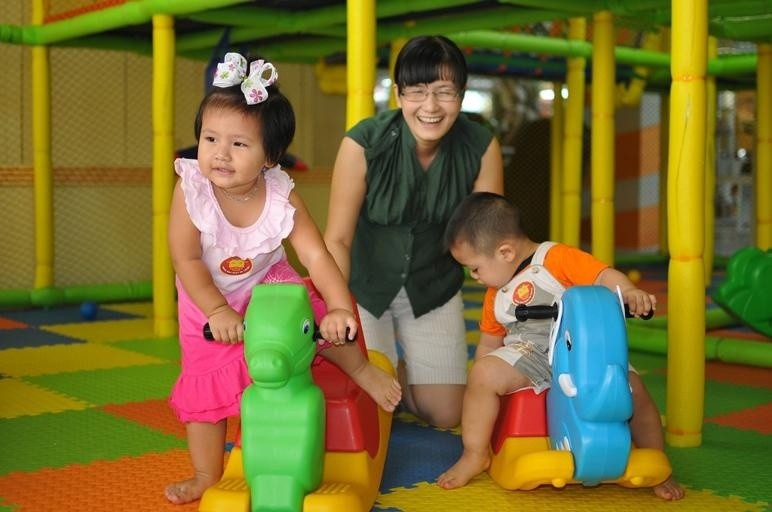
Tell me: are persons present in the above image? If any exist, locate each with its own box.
[163,48,404,506]
[433,191,688,502]
[323,34,506,430]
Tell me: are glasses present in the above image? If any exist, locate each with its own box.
[399,84,461,102]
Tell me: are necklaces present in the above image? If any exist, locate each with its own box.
[213,175,262,203]
